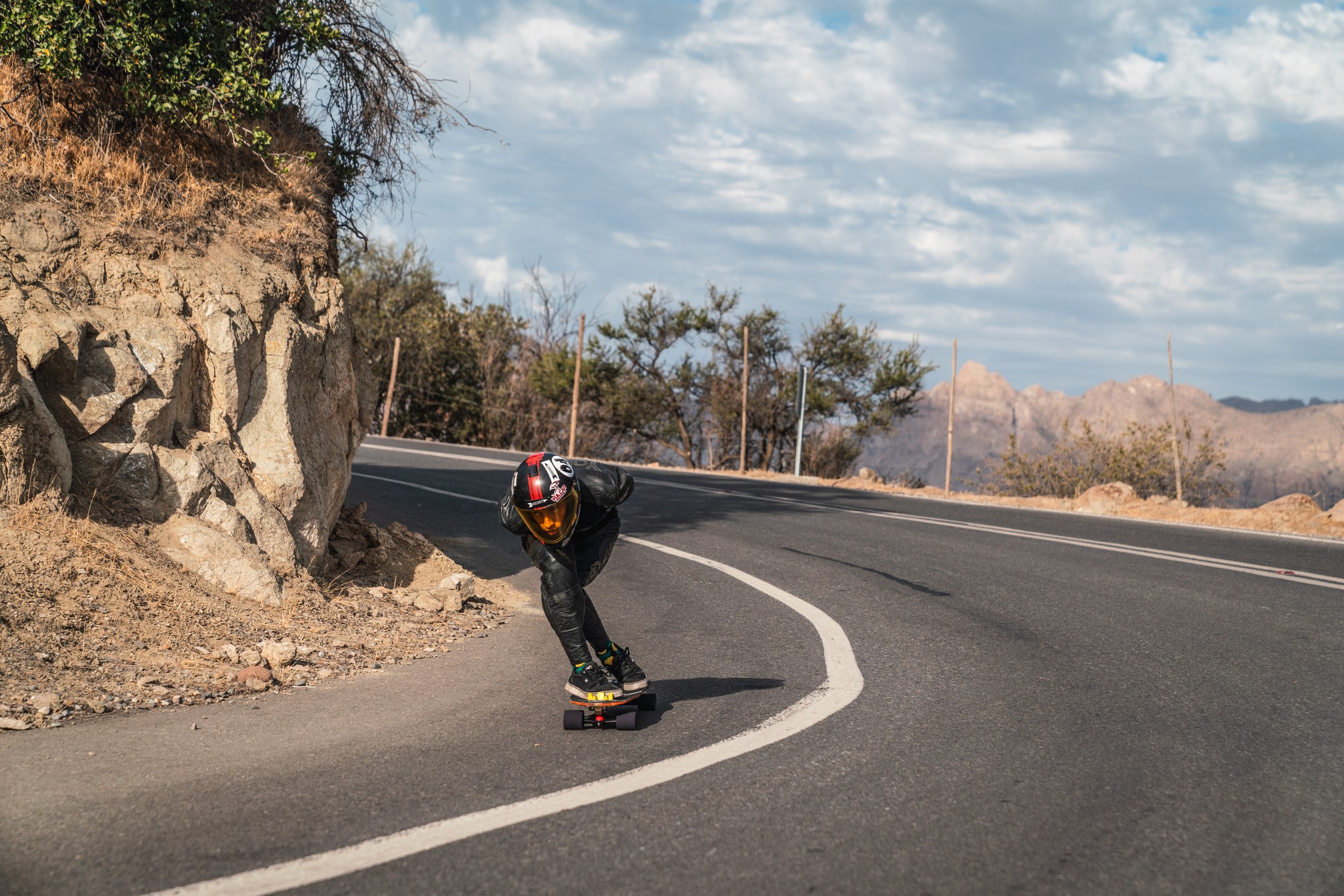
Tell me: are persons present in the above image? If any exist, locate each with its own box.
[498,452,649,700]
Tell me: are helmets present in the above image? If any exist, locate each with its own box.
[510,453,584,549]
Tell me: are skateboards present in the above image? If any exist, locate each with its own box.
[563,681,657,730]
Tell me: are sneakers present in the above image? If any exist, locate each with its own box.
[596,642,648,691]
[564,661,623,700]
